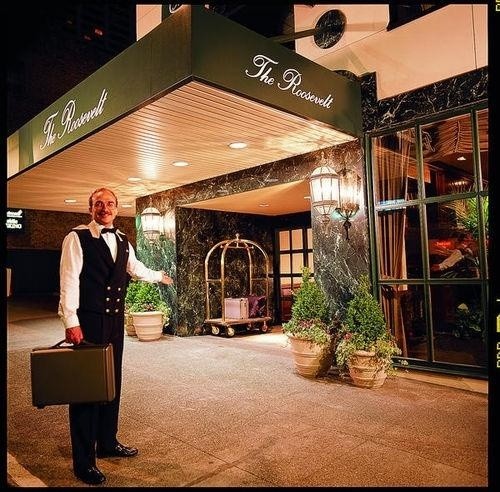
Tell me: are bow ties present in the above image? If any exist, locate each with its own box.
[101,227,118,234]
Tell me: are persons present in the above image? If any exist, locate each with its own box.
[429,226,481,273]
[29,187,174,488]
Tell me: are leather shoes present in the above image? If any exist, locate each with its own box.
[96,439,138,459]
[73,464,106,485]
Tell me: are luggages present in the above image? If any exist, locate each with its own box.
[223,297,249,319]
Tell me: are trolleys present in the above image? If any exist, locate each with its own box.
[203,234,271,337]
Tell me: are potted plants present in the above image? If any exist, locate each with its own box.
[124,277,173,341]
[282,267,409,390]
[439,182,489,365]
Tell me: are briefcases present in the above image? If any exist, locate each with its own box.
[28,338,116,410]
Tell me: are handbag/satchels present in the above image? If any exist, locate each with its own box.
[242,294,267,318]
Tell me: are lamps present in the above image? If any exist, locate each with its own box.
[309,150,340,226]
[140,197,162,247]
